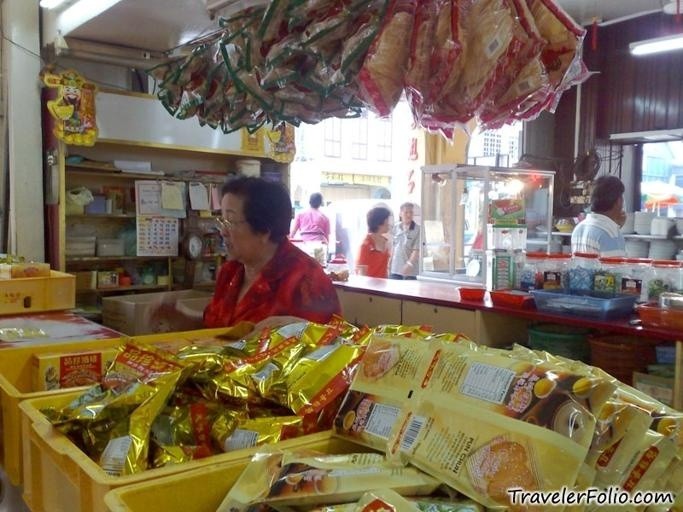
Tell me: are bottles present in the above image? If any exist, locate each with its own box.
[520,251,682,295]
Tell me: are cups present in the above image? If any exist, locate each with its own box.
[101,270,119,289]
[660,292,683,310]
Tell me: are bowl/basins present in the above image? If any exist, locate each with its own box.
[554,224,577,233]
[634,211,656,234]
[459,287,486,301]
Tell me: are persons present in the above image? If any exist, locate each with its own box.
[569,174,629,259]
[288,193,331,263]
[353,206,391,279]
[143,175,342,335]
[386,201,420,280]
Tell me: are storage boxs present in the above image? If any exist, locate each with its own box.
[1,259,506,512]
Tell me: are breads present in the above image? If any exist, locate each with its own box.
[480,441,541,512]
[364,340,392,378]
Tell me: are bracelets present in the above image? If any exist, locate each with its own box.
[405,260,414,267]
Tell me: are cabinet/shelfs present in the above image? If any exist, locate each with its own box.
[42,82,683,409]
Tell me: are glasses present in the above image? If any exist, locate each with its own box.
[212,213,250,229]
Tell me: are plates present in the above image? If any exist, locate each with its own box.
[625,239,675,259]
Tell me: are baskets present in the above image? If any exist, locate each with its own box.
[588,331,657,386]
[636,299,683,332]
[654,344,675,364]
[527,322,589,363]
[530,287,640,323]
